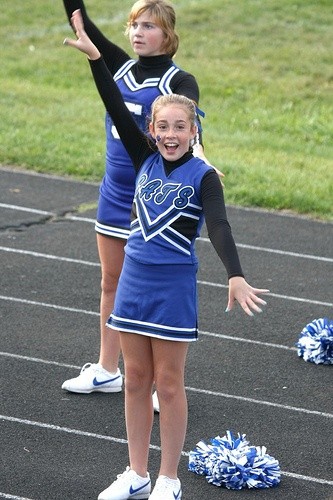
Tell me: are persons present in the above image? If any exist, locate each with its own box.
[63,8,271,500]
[58,0,226,412]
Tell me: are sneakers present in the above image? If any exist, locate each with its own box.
[151,391,161,413]
[62,362,123,393]
[98,466,151,500]
[148,475,182,500]
